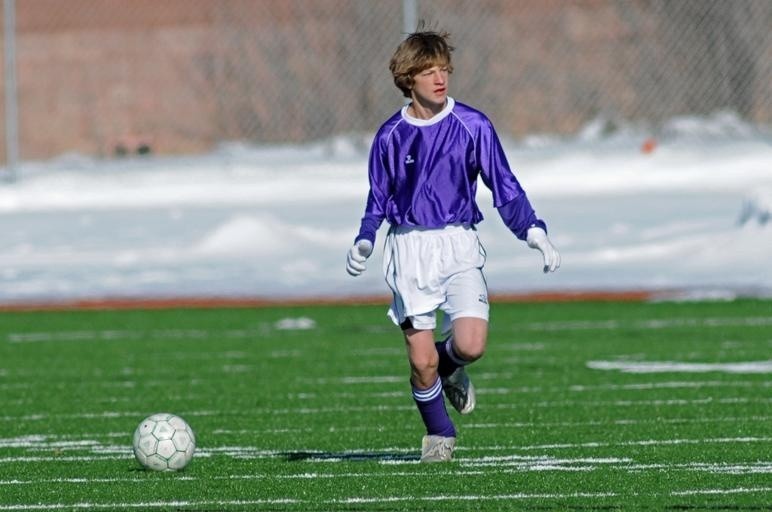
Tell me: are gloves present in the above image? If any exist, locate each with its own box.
[526,227,562,273]
[346,238,373,276]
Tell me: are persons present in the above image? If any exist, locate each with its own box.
[342,16,564,467]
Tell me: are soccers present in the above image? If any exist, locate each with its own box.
[132,412,194,474]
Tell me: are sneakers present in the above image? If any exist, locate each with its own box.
[420,433,456,463]
[434,340,477,414]
